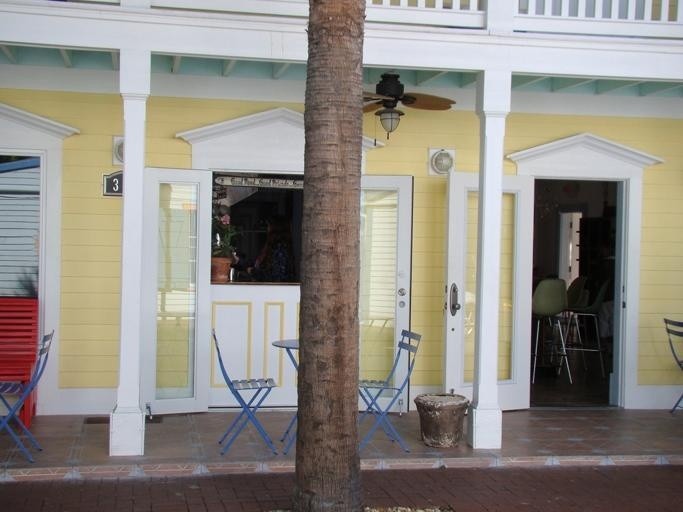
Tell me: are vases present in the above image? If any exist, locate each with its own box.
[413,393,468,450]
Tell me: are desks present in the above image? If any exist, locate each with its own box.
[270,336,304,458]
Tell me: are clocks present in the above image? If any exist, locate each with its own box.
[431,150,453,175]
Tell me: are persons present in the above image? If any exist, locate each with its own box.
[230,236,257,283]
[245,213,295,282]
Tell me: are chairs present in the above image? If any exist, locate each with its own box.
[352,328,426,456]
[204,325,278,461]
[531,274,608,388]
[660,318,683,414]
[2,332,59,461]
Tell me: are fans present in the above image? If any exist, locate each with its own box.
[360,74,456,119]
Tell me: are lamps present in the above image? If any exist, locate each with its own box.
[371,72,415,139]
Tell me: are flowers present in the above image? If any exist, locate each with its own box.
[211,207,240,285]
